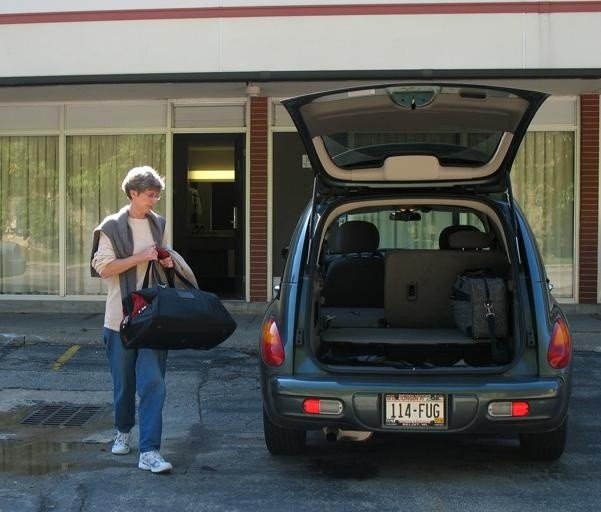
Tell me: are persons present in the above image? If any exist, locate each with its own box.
[89,164,174,475]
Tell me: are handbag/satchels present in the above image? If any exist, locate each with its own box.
[120,286,237,351]
[448,268,508,339]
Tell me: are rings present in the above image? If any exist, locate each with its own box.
[171,261,172,264]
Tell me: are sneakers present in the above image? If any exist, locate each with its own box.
[112,431,132,454]
[138,450,173,473]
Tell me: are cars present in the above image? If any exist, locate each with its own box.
[260,81,573,460]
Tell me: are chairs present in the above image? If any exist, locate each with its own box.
[320,220,510,329]
